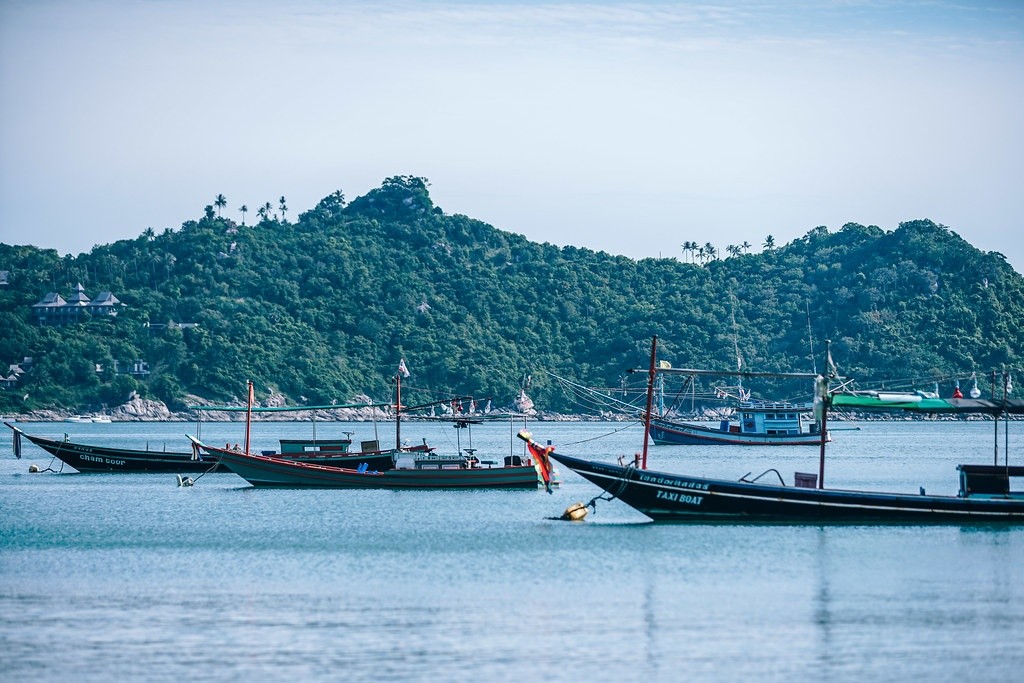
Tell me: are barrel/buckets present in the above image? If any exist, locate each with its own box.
[720,420,730,432]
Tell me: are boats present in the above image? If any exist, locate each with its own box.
[3,399,437,475]
[547,283,833,445]
[184,356,541,489]
[515,335,1024,526]
